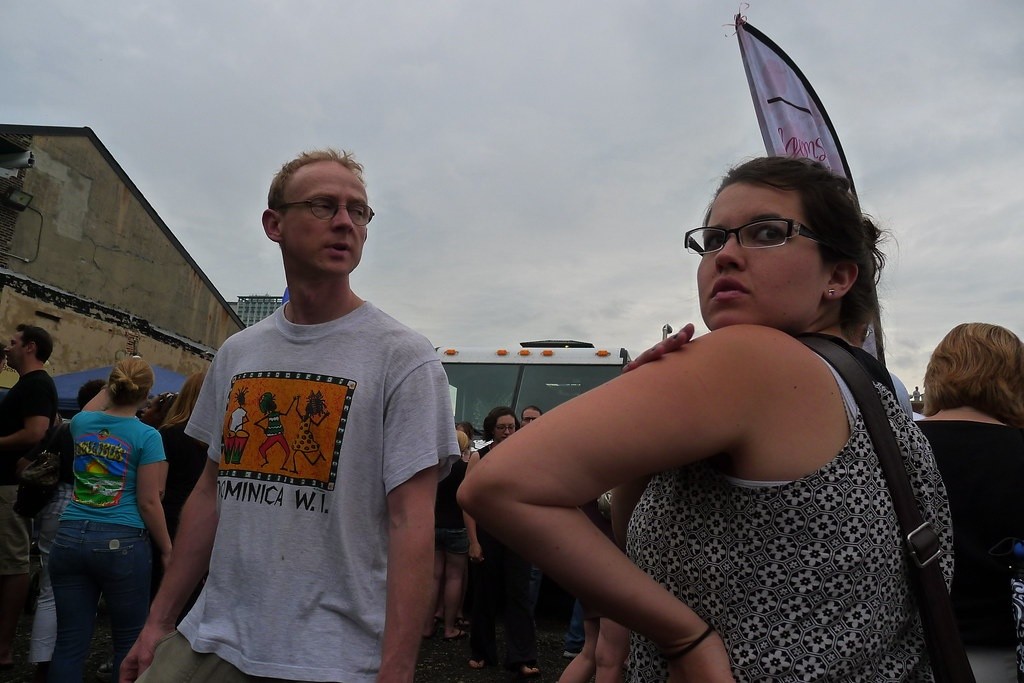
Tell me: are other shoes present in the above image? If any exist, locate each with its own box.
[469,659,486,671]
[520,665,541,680]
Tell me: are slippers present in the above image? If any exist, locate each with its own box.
[447,629,469,640]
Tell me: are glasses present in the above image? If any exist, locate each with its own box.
[495,424,516,431]
[523,417,536,421]
[684,217,819,256]
[277,196,375,226]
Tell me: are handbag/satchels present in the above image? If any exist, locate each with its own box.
[13,449,65,514]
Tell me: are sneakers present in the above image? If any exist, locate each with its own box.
[563,642,583,658]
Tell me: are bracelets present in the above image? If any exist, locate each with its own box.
[665,623,716,662]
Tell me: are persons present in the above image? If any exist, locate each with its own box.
[909,321,1023,683]
[455,152,954,683]
[96,371,916,683]
[0,323,59,668]
[120,148,454,683]
[841,218,912,423]
[28,377,108,683]
[44,353,173,683]
